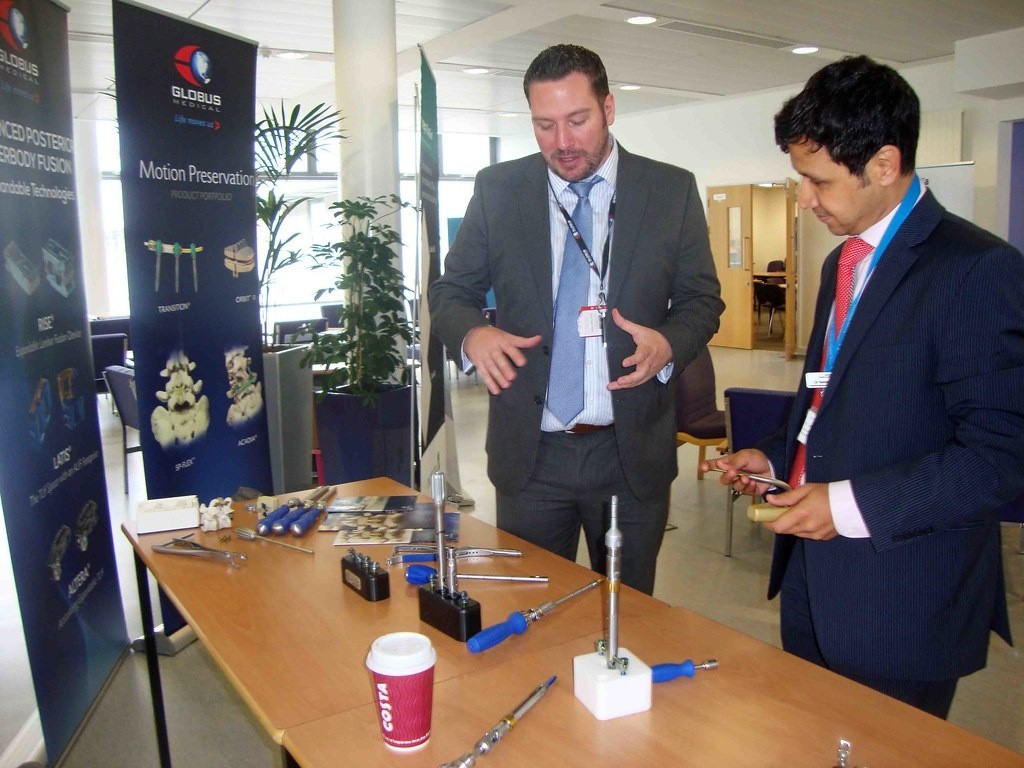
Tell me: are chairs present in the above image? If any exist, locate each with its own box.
[90,333,128,414]
[89,318,132,350]
[102,365,141,494]
[675,346,727,480]
[754,261,784,338]
[723,387,797,556]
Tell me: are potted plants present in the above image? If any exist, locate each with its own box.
[288,193,421,490]
[251,96,352,496]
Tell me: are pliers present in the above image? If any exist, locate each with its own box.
[388,545,523,565]
[152,537,247,568]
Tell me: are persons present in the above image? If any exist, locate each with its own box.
[700,55,1024,722]
[428,43,723,596]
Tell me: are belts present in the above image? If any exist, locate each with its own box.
[569,425,613,433]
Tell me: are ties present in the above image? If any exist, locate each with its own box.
[787,237,874,492]
[546,174,605,426]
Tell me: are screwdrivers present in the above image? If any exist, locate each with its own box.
[465,579,603,654]
[256,496,300,536]
[404,565,549,585]
[441,674,556,768]
[271,486,328,536]
[289,485,336,538]
[650,659,719,682]
[236,528,314,554]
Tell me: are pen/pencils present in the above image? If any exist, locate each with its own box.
[707,467,790,489]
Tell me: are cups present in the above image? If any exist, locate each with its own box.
[366,632,436,753]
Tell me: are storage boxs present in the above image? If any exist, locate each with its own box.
[136,495,199,535]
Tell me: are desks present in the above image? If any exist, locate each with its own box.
[752,272,797,311]
[121,476,1024,768]
